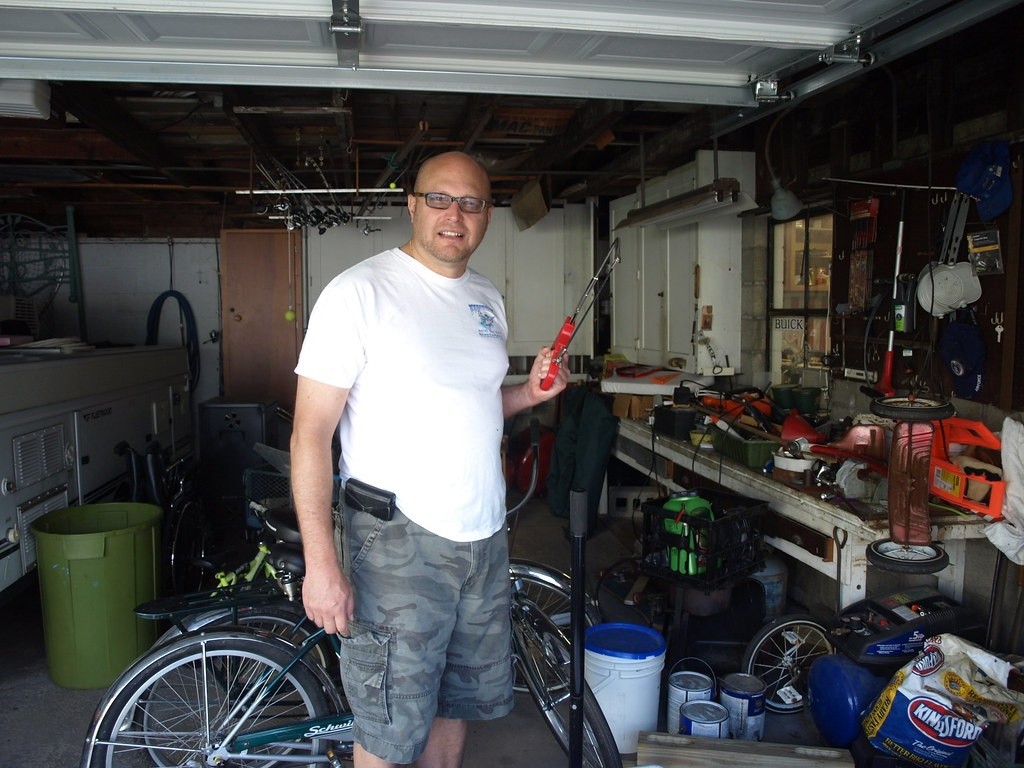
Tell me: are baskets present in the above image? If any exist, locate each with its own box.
[242,462,341,527]
[708,422,782,467]
[640,487,770,593]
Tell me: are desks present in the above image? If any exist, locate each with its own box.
[597,415,1002,610]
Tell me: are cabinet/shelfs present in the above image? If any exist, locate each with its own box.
[306,202,597,386]
[784,212,834,298]
[610,150,758,374]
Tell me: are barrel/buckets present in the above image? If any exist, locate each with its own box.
[583,623,766,754]
[663,491,723,576]
[27,501,165,689]
[769,383,822,413]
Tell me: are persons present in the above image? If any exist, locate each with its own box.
[287,151,572,768]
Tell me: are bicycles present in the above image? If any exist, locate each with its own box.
[78,407,626,767]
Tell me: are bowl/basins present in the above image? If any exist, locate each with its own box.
[690,429,712,446]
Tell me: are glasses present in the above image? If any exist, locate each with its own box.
[413,192,492,214]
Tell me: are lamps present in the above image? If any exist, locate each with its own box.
[610,105,759,231]
[763,105,803,220]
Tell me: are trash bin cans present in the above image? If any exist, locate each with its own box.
[29,502,165,690]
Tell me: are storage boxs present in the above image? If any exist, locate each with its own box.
[706,421,785,469]
[640,486,770,595]
